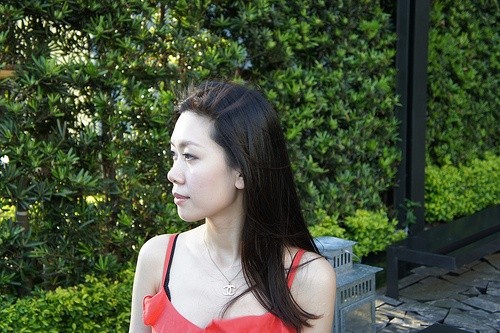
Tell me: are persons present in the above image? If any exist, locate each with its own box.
[127,79,336,333]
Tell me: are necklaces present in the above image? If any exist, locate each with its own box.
[202,223,246,296]
